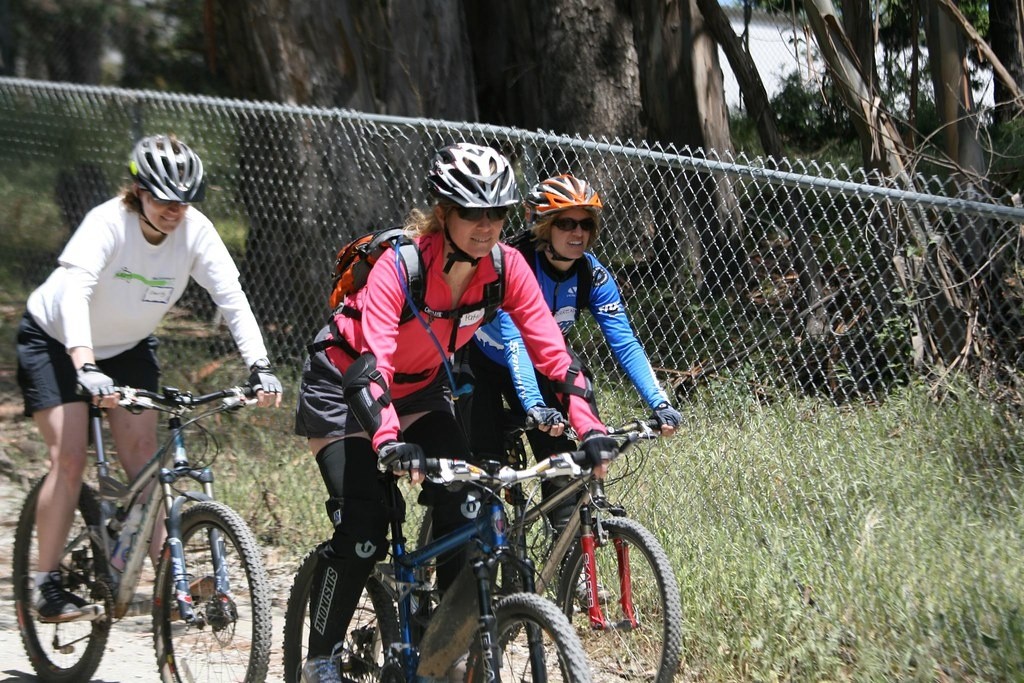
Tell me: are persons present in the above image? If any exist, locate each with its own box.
[13,134,284,619]
[462,173,681,616]
[291,146,617,683]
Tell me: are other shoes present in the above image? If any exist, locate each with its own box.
[301,642,350,682]
[574,569,614,608]
[171,573,217,622]
[35,569,83,623]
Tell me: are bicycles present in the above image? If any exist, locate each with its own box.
[282,414,681,683]
[11,384,273,683]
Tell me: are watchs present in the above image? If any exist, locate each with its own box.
[250,358,273,372]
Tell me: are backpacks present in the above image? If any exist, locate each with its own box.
[330,225,426,325]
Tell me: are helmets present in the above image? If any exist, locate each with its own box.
[427,142,519,207]
[126,135,207,203]
[524,174,602,224]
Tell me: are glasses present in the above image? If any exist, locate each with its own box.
[444,205,510,220]
[550,216,595,230]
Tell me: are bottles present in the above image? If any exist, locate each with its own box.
[109,504,146,573]
